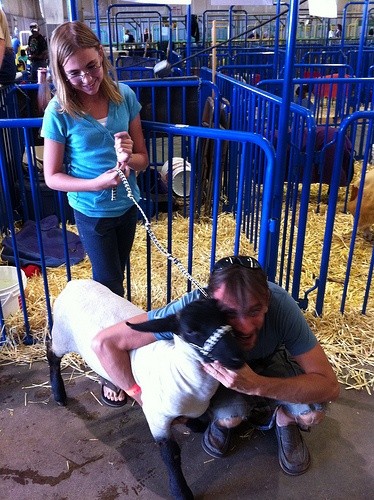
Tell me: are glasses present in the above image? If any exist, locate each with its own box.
[64,55,103,83]
[212,255,262,276]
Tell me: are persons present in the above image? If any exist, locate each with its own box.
[141,28,151,40]
[328,24,342,44]
[124,29,133,40]
[40,21,149,407]
[296,65,320,99]
[0,9,49,85]
[91,256,339,476]
[368,28,373,36]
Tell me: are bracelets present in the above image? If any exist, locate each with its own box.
[125,385,140,395]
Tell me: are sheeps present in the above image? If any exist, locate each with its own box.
[47,279,246,500]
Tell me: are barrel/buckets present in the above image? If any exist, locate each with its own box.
[0,265,27,319]
[160,156,191,197]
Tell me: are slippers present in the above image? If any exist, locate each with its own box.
[100,378,127,407]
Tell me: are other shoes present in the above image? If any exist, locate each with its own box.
[274,417,312,475]
[202,419,249,459]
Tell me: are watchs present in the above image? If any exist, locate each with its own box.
[251,68,261,81]
